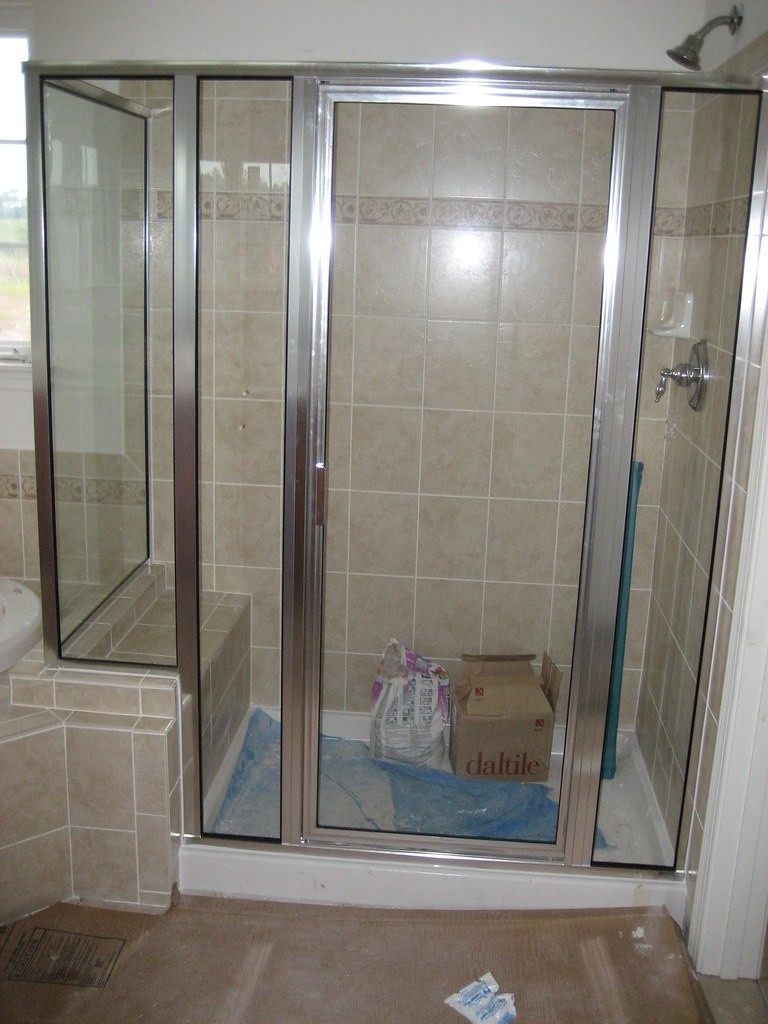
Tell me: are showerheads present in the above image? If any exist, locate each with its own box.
[663,0,743,73]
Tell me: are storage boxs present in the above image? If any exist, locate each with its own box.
[448,651,563,782]
